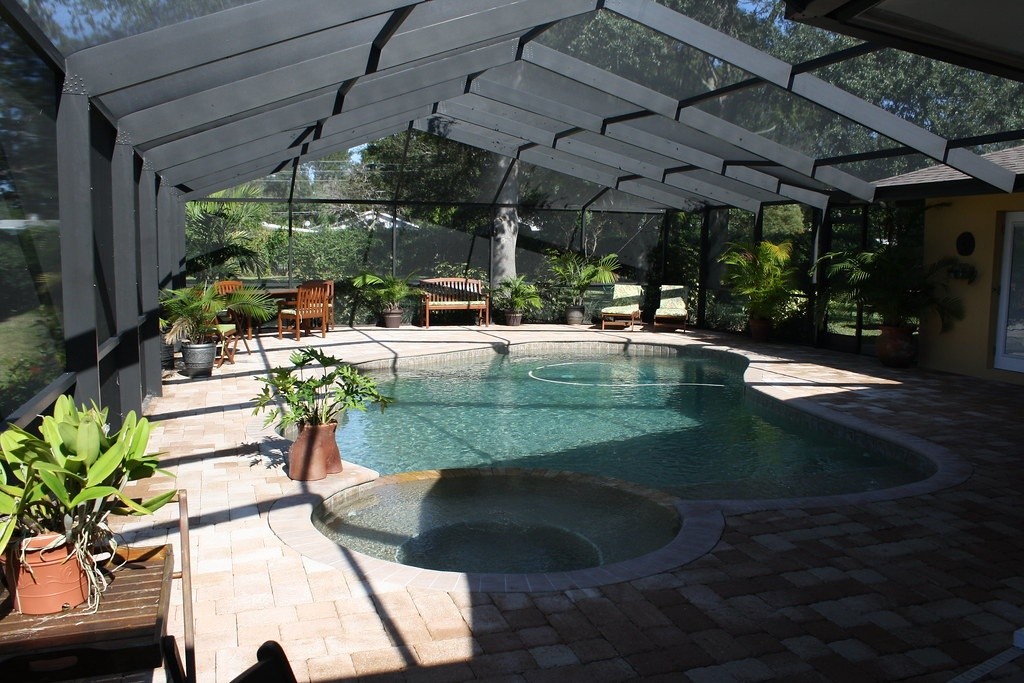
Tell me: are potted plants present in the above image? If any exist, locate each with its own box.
[808,245,977,368]
[349,268,428,328]
[255,344,399,481]
[0,394,177,615]
[716,238,802,339]
[544,249,623,326]
[159,281,278,379]
[484,273,543,326]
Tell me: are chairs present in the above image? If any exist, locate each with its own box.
[600,284,645,332]
[654,285,690,333]
[191,280,261,368]
[0,635,300,683]
[276,280,334,341]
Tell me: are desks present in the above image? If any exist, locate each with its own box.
[237,288,298,340]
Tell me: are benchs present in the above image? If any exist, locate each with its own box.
[419,278,490,327]
